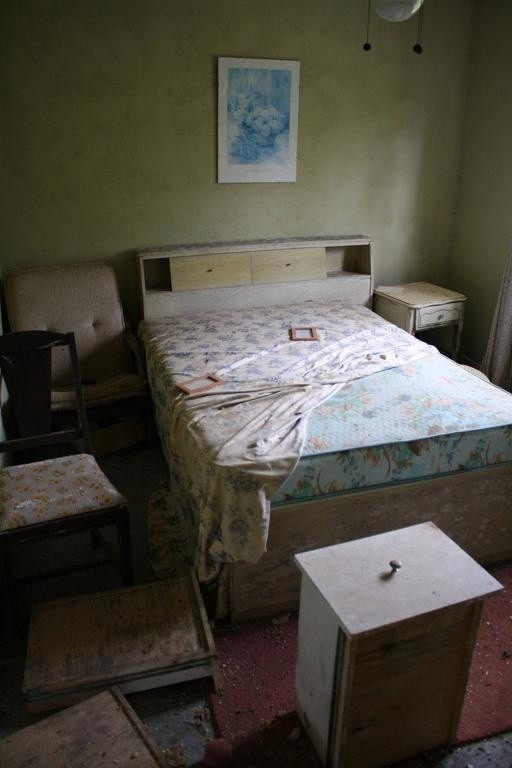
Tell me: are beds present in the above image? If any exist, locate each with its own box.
[128,234,512,629]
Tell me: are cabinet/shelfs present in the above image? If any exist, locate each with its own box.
[284,517,504,766]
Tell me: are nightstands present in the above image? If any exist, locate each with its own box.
[375,278,467,367]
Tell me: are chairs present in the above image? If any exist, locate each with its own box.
[5,262,154,455]
[0,327,157,617]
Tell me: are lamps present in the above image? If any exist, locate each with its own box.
[368,0,423,23]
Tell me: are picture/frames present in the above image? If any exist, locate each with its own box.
[173,369,221,396]
[286,325,318,343]
[216,56,300,184]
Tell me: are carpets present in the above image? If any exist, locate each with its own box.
[195,556,510,766]
